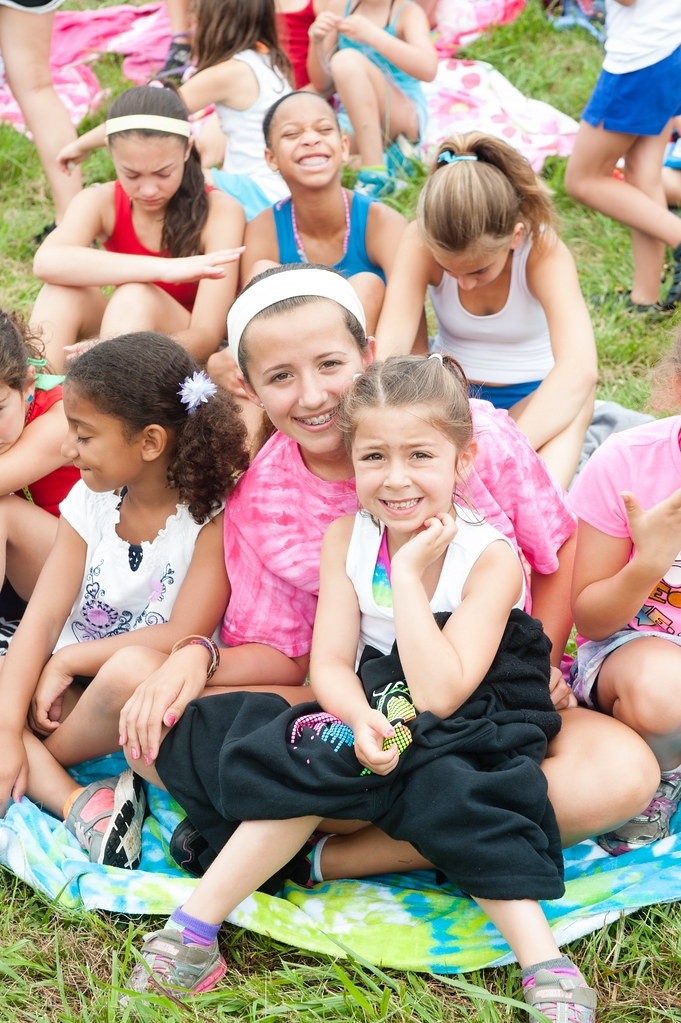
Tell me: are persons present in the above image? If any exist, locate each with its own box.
[0,0,681,602]
[0,331,252,870]
[117,264,662,893]
[126,355,595,1023]
[566,325,681,856]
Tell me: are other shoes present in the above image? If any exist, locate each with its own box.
[152,40,190,84]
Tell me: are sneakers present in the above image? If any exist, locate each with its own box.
[597,772,681,855]
[64,769,146,868]
[118,928,229,1016]
[519,958,600,1022]
[169,817,321,898]
[662,242,680,311]
[350,168,396,200]
[586,289,661,320]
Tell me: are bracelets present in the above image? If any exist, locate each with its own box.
[168,634,220,685]
